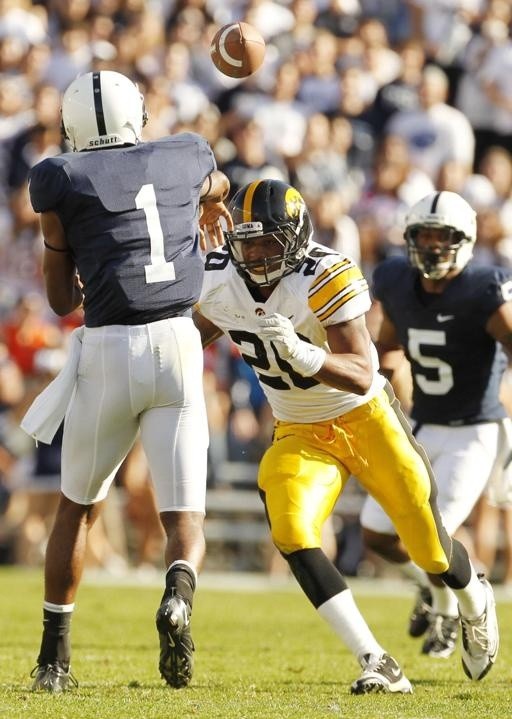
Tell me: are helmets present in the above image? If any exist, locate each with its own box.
[230,181,315,287]
[58,66,147,152]
[404,189,479,281]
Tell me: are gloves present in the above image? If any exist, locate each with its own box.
[255,312,325,380]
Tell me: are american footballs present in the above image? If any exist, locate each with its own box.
[209,23,267,79]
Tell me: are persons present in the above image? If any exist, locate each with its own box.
[365,190,512,659]
[192,179,500,696]
[29,69,235,692]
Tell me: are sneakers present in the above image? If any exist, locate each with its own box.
[347,651,414,697]
[409,585,434,638]
[458,579,499,682]
[29,659,78,695]
[154,587,193,689]
[420,603,461,658]
